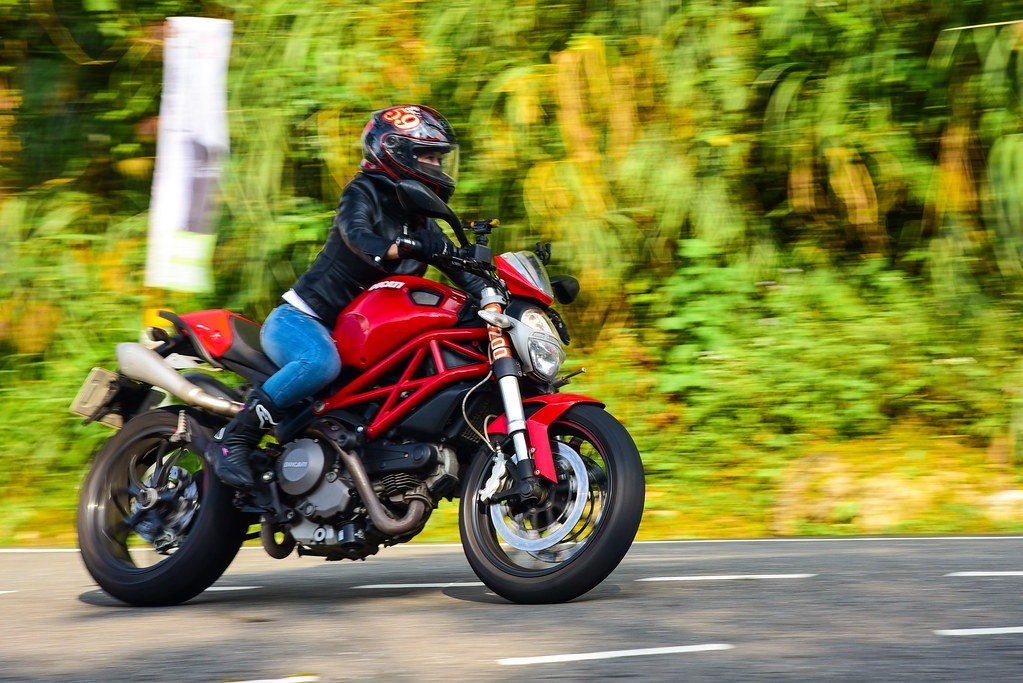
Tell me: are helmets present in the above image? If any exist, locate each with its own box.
[360,104,458,204]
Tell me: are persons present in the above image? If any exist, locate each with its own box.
[199,103,493,491]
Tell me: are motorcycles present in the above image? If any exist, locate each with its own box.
[68,176,645,607]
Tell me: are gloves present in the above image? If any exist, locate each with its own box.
[397,230,453,264]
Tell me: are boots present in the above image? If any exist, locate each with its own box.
[204,384,287,489]
